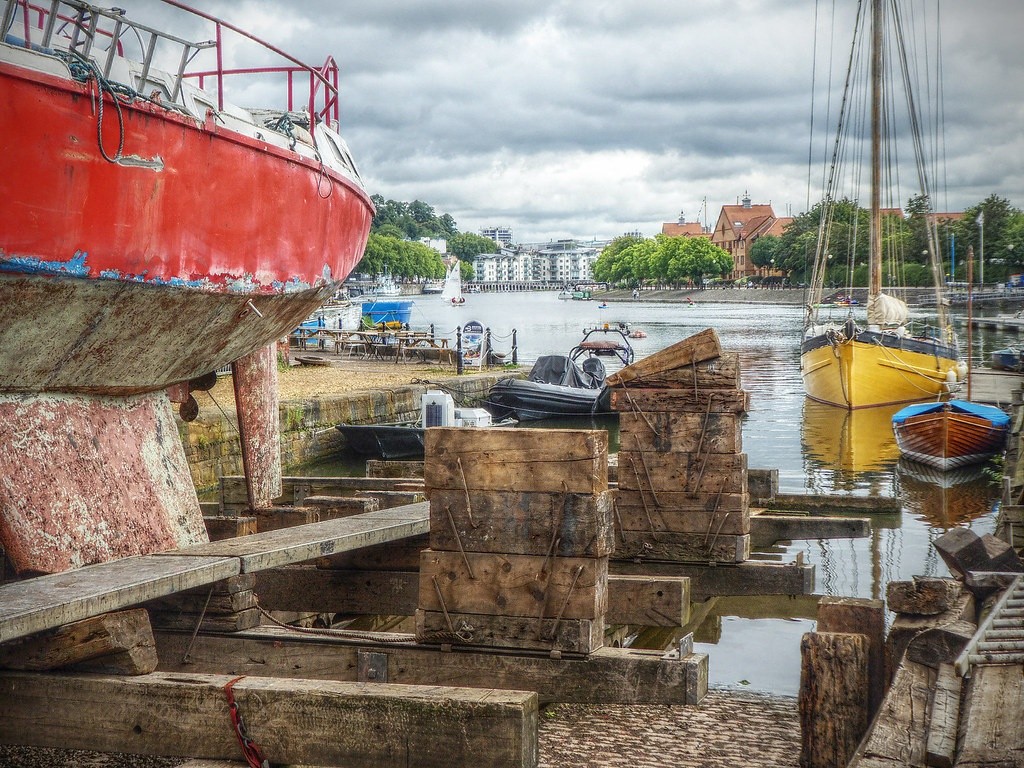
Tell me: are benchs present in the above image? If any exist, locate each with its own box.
[392,345,454,365]
[331,339,370,359]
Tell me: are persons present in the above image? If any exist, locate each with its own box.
[632,288,638,299]
[603,301,607,306]
[451,296,466,303]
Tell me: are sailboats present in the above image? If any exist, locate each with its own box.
[889,248,1013,472]
[804,394,950,605]
[442,259,468,307]
[798,0,972,411]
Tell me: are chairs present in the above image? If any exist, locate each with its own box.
[407,332,427,360]
[359,333,378,360]
[394,332,410,360]
[365,332,391,362]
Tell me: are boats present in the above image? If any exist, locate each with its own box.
[894,455,996,534]
[453,320,493,366]
[489,324,636,424]
[598,305,609,309]
[0,0,378,395]
[558,287,573,300]
[423,283,444,295]
[965,346,1024,406]
[333,390,520,459]
[290,283,415,346]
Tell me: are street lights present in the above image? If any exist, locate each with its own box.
[922,249,928,288]
[827,254,832,289]
[770,259,775,285]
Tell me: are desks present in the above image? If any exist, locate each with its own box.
[289,326,452,366]
